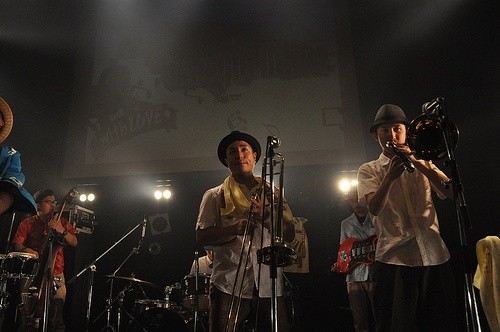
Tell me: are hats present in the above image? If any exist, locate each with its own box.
[218,130,261,167]
[0,97,13,142]
[370,104,411,133]
[33,188,55,203]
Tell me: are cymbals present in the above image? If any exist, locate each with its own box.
[106,274,161,288]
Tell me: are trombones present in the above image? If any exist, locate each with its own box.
[222,151,297,332]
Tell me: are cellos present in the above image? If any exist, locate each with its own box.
[14,185,80,332]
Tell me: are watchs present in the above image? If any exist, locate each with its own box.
[61,228,68,237]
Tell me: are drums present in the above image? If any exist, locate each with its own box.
[133,298,178,332]
[5,250,40,303]
[182,272,212,312]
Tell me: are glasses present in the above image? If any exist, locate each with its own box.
[39,200,58,204]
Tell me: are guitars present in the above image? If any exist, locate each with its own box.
[336,232,378,275]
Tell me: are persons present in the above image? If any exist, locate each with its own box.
[0,95,36,217]
[356,103,458,332]
[195,130,296,332]
[189,246,215,277]
[10,187,79,332]
[331,184,378,332]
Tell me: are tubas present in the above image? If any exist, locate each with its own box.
[386,111,460,173]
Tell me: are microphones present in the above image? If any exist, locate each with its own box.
[422,97,443,112]
[267,136,281,148]
[142,218,147,240]
